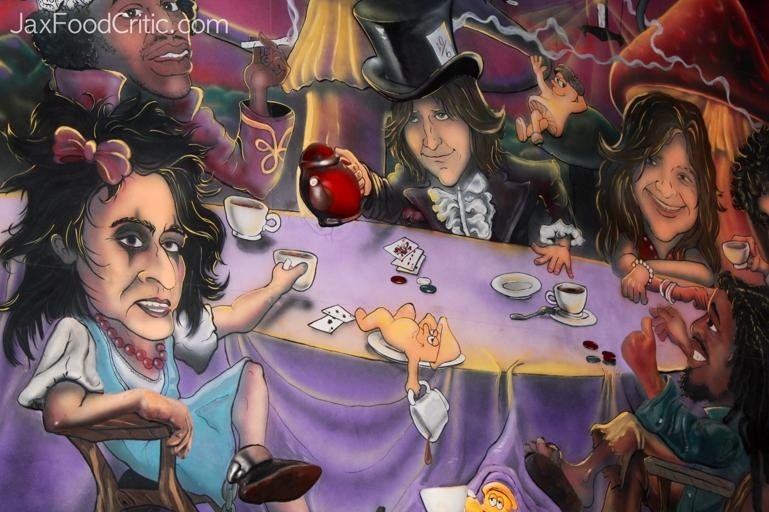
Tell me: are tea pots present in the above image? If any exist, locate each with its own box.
[298,143,366,228]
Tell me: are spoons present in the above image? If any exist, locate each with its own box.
[509,306,557,321]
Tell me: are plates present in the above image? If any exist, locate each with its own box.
[490,271,543,300]
[549,304,598,327]
[368,330,466,369]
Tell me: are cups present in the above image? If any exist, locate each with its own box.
[420,485,475,512]
[273,249,318,291]
[722,240,750,269]
[406,380,451,444]
[545,283,587,317]
[224,195,281,240]
